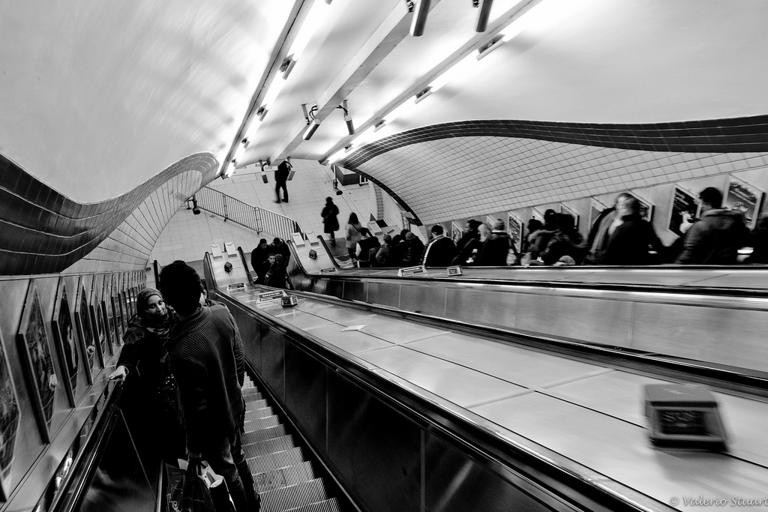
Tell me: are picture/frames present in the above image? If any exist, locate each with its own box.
[451,173,767,255]
[0,269,147,503]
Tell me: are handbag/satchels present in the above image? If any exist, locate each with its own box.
[170,455,237,512]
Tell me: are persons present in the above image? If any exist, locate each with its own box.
[269,236,291,272]
[250,238,273,279]
[254,255,275,284]
[269,254,288,288]
[106,257,262,512]
[272,160,290,203]
[342,185,767,270]
[321,196,341,249]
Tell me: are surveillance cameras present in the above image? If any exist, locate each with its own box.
[261,171,268,183]
[345,115,355,136]
[332,178,342,195]
[302,118,321,140]
[474,0,494,33]
[193,207,200,214]
[410,0,430,37]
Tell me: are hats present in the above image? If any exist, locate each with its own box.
[699,188,724,208]
[136,286,162,311]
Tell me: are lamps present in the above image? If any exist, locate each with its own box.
[218,0,504,181]
